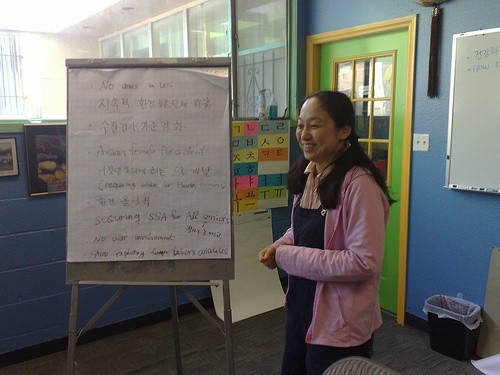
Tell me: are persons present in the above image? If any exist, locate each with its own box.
[259,90,398,375]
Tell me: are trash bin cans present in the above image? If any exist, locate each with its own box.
[422,294,483,362]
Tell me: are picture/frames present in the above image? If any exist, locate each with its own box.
[24,123,67,196]
[0,137,19,177]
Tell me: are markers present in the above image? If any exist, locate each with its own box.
[480,188,498,192]
[452,185,475,189]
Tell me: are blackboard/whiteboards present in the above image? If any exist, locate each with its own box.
[444,27,499,194]
